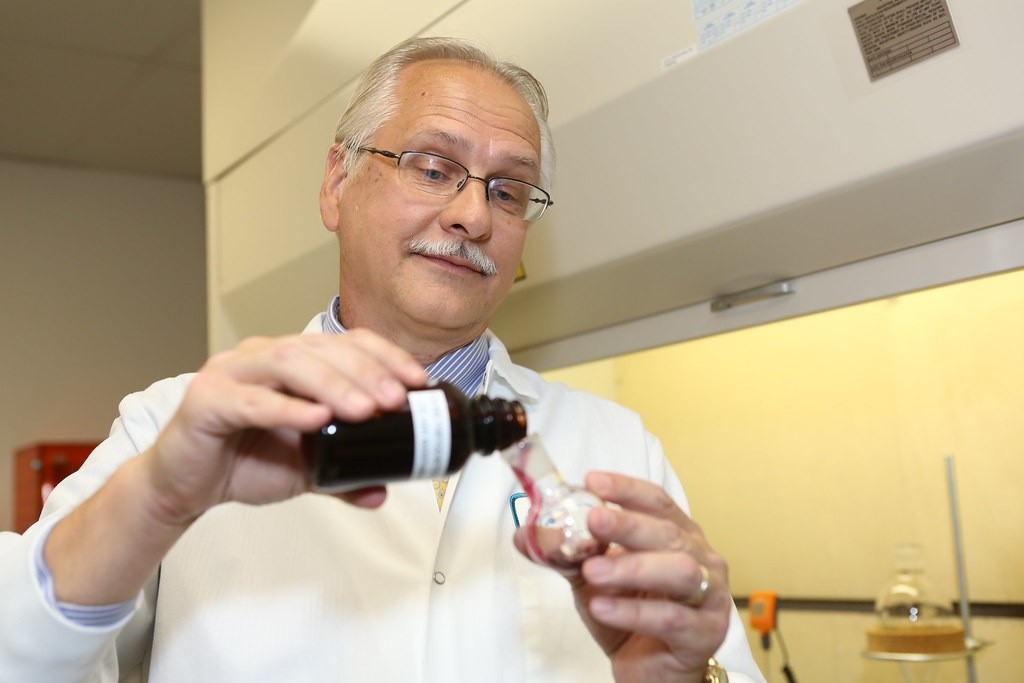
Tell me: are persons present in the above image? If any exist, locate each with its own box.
[0,36,766,683]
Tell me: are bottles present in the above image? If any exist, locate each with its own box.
[876,541,954,628]
[302,378,528,495]
[499,430,610,589]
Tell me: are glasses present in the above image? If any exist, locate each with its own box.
[347,145,555,222]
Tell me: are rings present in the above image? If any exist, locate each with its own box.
[683,563,713,609]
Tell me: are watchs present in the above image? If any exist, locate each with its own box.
[702,654,729,683]
